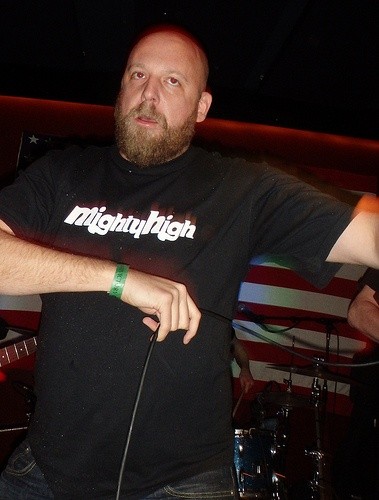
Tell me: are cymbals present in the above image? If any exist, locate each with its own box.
[260,391,315,408]
[267,363,358,386]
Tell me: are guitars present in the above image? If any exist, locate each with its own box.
[0,336,40,383]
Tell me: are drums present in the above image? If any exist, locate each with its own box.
[234,428,287,498]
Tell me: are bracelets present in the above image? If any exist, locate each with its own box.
[108,263,129,300]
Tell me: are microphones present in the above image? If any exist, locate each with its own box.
[236,302,268,331]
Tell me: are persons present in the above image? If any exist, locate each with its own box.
[0,29,379,500]
[347,267,378,500]
[231,327,253,394]
